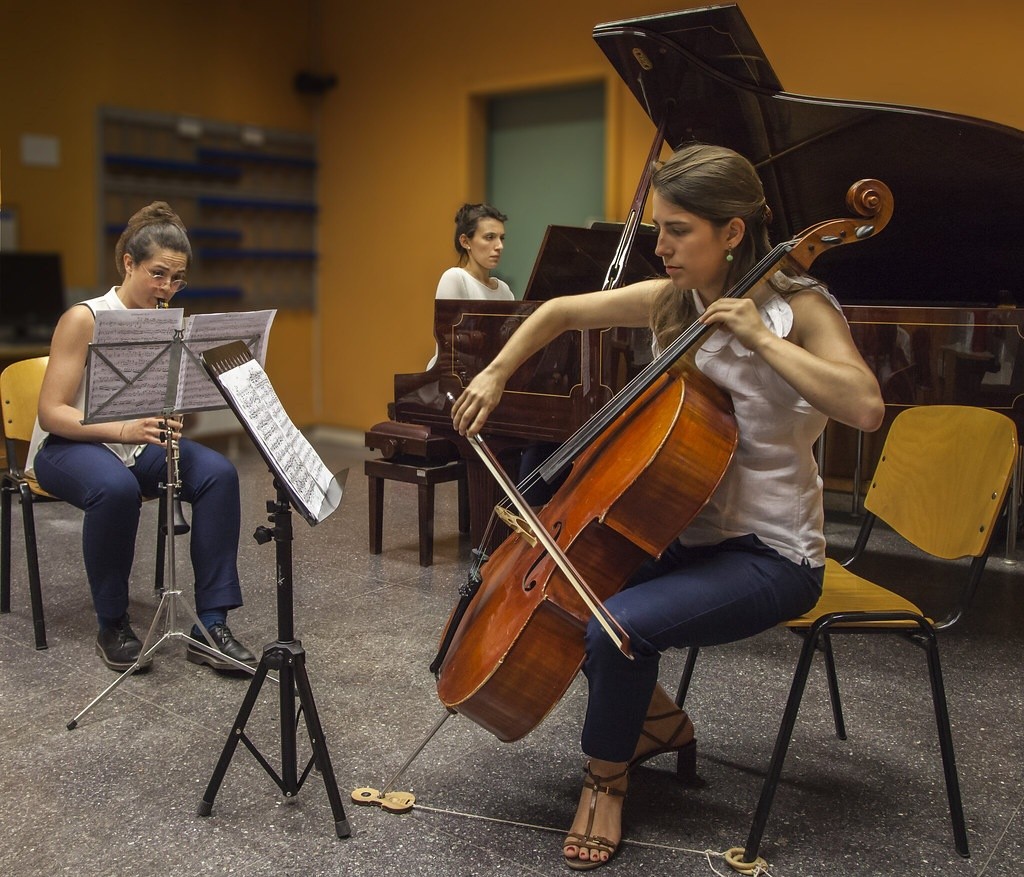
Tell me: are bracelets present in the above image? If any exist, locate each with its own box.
[120,423,127,445]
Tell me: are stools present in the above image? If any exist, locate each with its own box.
[364,419,472,568]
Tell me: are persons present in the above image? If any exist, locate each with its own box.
[422,202,573,530]
[449,138,886,869]
[26,199,261,673]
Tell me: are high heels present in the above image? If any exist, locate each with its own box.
[583,710,699,775]
[562,761,628,869]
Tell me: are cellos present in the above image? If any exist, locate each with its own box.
[429,177,895,744]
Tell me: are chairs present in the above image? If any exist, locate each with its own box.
[0,355,167,651]
[672,405,1021,864]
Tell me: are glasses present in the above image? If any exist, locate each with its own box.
[140,263,187,291]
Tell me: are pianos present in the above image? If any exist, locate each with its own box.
[392,3,1023,568]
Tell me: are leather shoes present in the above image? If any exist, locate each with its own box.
[94,623,153,670]
[187,622,259,669]
